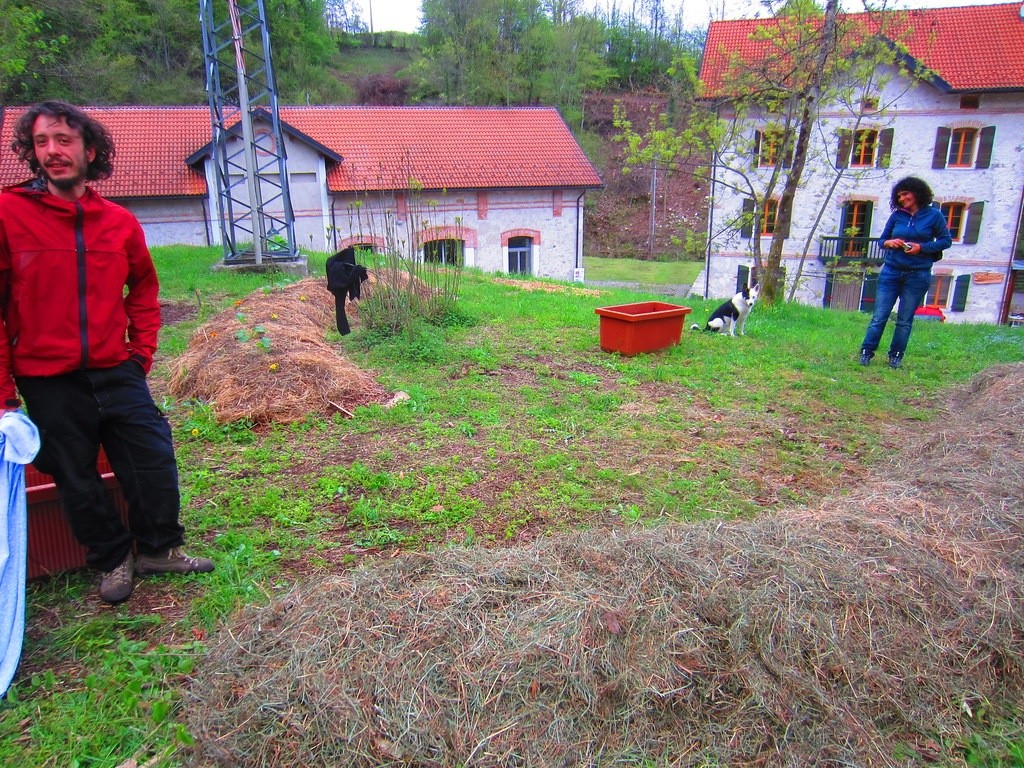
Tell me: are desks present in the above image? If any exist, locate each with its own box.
[1007,318,1024,327]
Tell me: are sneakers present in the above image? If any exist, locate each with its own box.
[136,545,215,577]
[100,547,135,601]
[890,351,902,366]
[860,348,874,365]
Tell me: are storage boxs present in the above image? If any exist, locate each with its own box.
[594,300,692,359]
[25,442,135,583]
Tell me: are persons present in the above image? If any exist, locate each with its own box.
[0,99,214,605]
[859,176,952,367]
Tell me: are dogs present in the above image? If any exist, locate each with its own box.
[689,282,760,338]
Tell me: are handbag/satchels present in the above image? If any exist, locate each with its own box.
[931,250,942,262]
